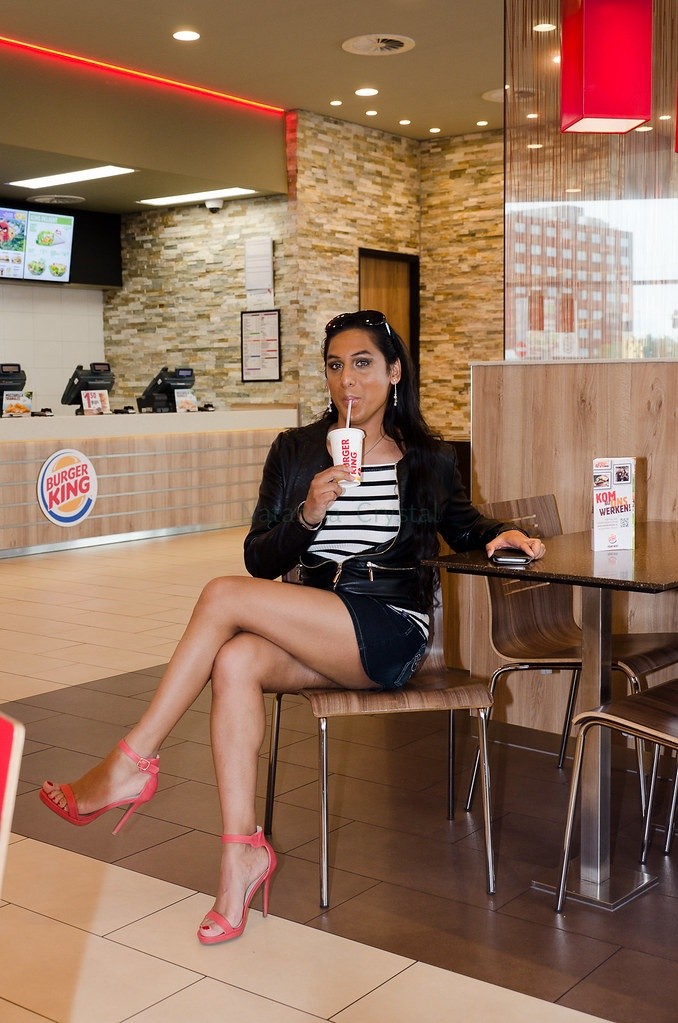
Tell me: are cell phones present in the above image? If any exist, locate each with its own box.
[494,557,527,563]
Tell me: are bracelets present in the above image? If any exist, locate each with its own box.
[301,504,320,526]
[363,435,386,456]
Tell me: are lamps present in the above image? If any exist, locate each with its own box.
[559,0,653,135]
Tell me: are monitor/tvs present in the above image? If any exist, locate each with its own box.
[0,371,26,410]
[61,369,114,405]
[142,372,195,397]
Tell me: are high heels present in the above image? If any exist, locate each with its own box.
[197,825,277,944]
[39,738,160,836]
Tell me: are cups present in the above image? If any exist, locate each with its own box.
[327,428,365,487]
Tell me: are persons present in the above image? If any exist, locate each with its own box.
[39,310,545,945]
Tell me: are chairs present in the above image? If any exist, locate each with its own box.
[553,677,678,914]
[265,565,495,909]
[463,493,678,831]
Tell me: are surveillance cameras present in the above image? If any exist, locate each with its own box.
[205,200,223,213]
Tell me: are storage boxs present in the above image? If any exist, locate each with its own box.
[591,457,648,554]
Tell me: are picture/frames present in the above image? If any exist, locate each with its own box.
[241,309,281,383]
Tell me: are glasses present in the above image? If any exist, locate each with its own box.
[325,310,392,335]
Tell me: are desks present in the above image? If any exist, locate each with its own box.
[420,519,678,912]
